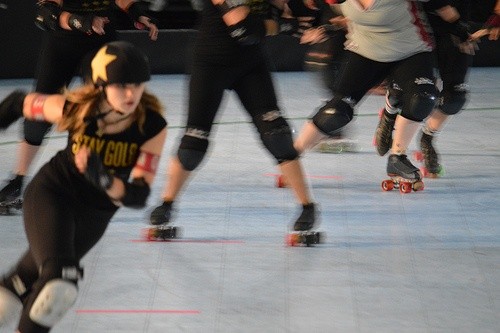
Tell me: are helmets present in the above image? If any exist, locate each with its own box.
[90,41,150,86]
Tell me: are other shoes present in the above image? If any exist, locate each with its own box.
[318,139,358,153]
[0,174,24,215]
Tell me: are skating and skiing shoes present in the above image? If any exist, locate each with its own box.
[285,203,321,248]
[415,125,437,179]
[146,200,176,242]
[276,174,287,188]
[374,107,398,158]
[381,154,424,193]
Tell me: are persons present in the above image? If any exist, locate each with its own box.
[1,42,168,333]
[0,0,159,218]
[279,0,500,193]
[148,0,322,246]
